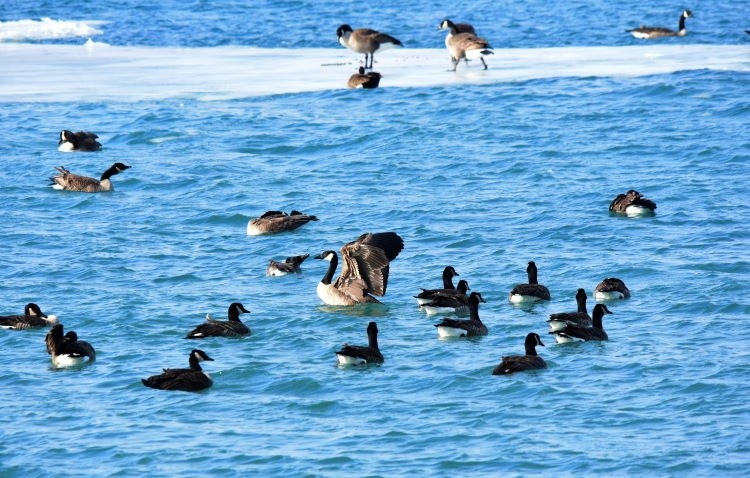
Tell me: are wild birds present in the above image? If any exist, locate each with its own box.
[335,24,403,69]
[48,162,132,192]
[440,19,494,72]
[58,130,102,151]
[609,189,657,212]
[0,208,630,392]
[347,66,384,90]
[626,8,692,39]
